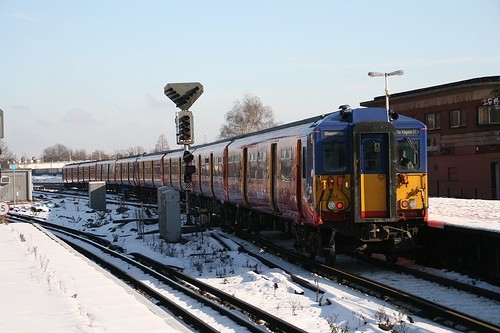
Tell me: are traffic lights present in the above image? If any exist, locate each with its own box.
[179,115,192,141]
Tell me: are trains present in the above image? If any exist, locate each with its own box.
[60,105,429,264]
